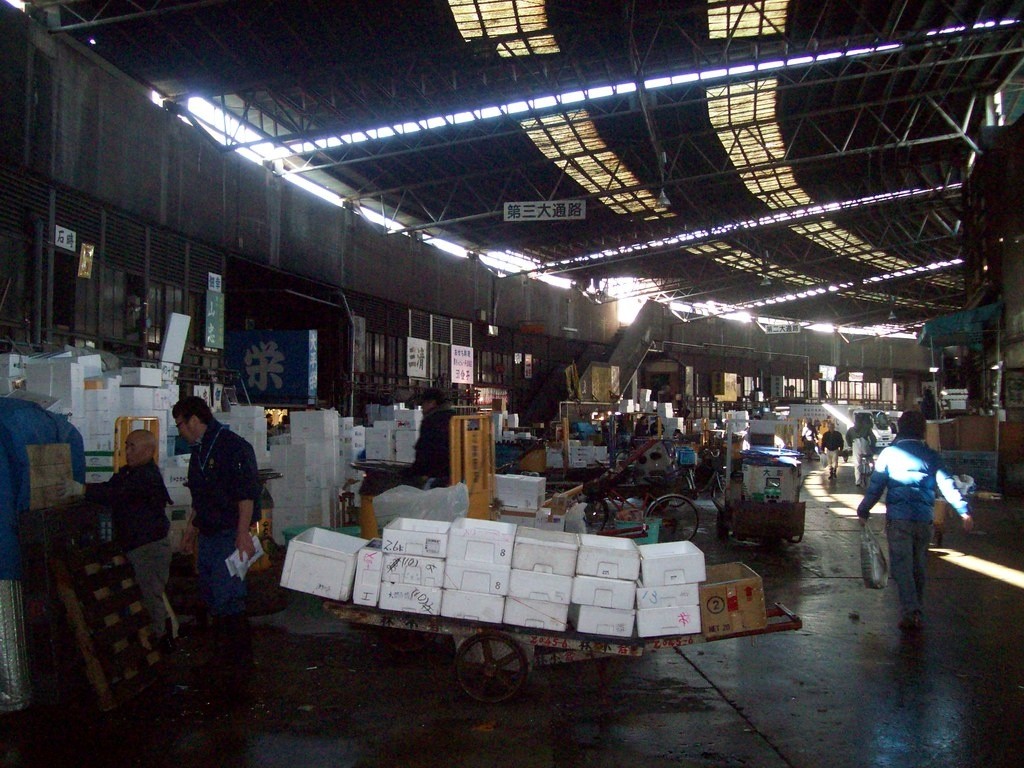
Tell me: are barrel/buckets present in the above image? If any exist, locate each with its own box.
[614,517,662,546]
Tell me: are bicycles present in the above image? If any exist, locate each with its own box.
[860,450,875,486]
[583,438,726,544]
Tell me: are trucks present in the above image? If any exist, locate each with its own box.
[841,405,894,448]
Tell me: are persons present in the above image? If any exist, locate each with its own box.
[857,410,975,632]
[846,417,877,486]
[921,389,941,420]
[821,423,844,479]
[174,395,261,703]
[404,387,458,491]
[635,415,665,437]
[53,430,174,659]
[801,418,819,463]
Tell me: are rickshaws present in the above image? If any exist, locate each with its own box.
[319,594,802,703]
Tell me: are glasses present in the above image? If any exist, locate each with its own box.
[176,420,184,428]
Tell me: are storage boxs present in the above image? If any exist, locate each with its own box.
[0,349,997,638]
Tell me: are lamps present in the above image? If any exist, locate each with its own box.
[888,309,897,319]
[760,270,771,286]
[655,169,672,207]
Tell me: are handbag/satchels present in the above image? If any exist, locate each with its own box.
[820,452,828,469]
[860,520,887,589]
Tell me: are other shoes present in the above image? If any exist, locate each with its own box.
[828,476,833,479]
[895,611,925,631]
[834,473,837,478]
